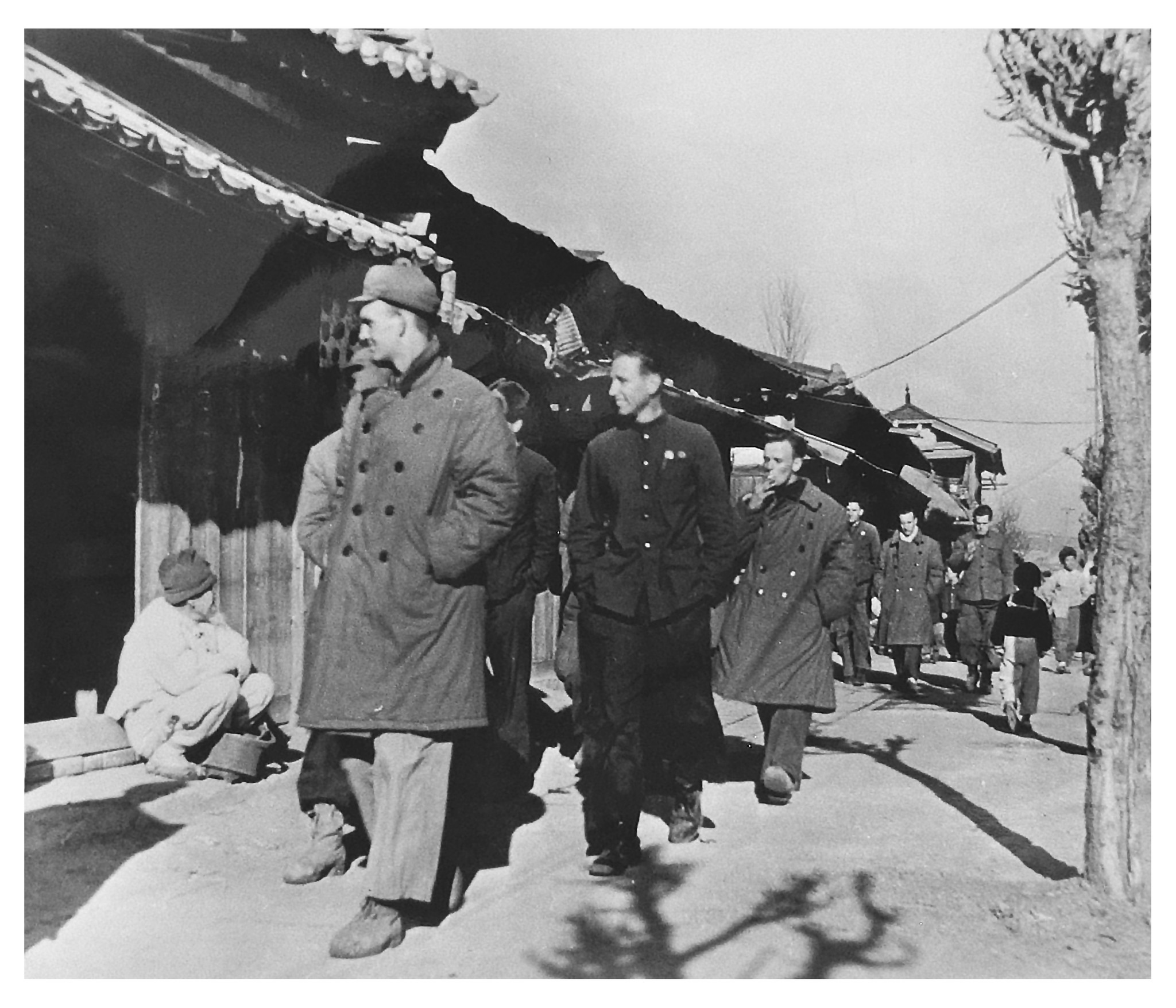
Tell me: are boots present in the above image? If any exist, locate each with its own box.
[283,803,346,884]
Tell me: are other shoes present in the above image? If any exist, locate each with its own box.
[965,671,979,691]
[896,676,917,696]
[1004,701,1031,734]
[763,765,793,804]
[1083,660,1093,676]
[843,667,865,686]
[145,745,197,781]
[1055,661,1071,675]
[980,678,992,694]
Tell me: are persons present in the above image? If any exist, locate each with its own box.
[568,344,741,874]
[303,262,524,961]
[284,333,1100,888]
[105,547,276,783]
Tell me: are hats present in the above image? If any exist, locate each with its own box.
[158,548,217,605]
[347,265,441,317]
[485,377,530,423]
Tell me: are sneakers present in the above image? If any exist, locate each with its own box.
[668,793,703,845]
[588,836,640,876]
[329,896,402,959]
